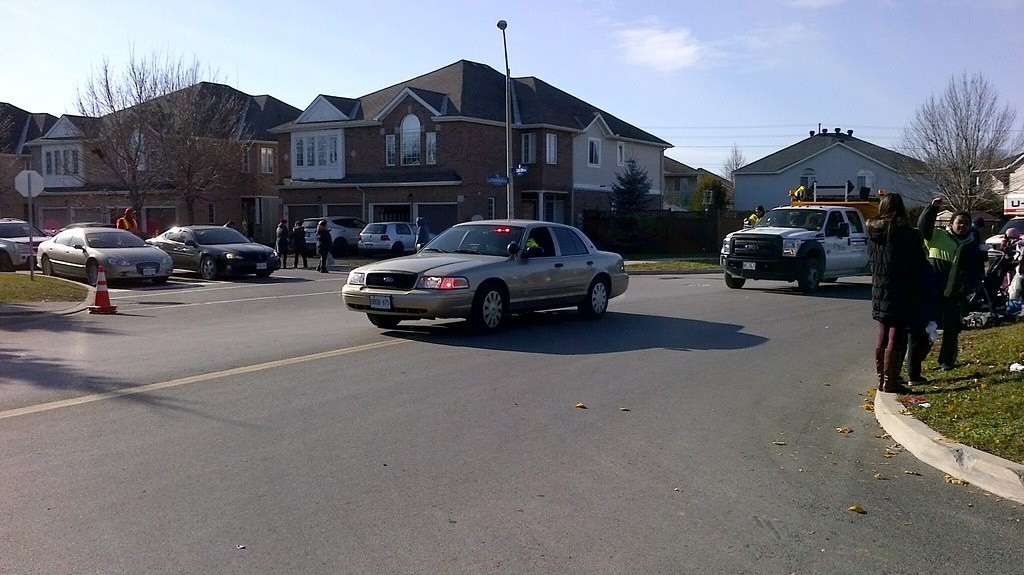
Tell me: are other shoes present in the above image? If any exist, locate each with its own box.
[280,265,329,273]
[908,376,927,386]
[941,358,956,371]
[903,359,908,366]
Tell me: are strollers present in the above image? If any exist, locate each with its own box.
[963,247,1021,331]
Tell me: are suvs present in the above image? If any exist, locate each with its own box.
[0,217,54,272]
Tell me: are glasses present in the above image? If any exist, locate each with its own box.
[756,211,761,213]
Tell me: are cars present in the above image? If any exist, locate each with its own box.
[145,225,281,281]
[342,219,630,336]
[302,216,368,257]
[52,222,117,240]
[37,228,174,287]
[357,222,435,258]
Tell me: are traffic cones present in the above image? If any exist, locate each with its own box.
[86,266,118,314]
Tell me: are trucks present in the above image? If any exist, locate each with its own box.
[984,192,1024,246]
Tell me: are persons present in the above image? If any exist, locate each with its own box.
[276,218,332,272]
[414,217,429,250]
[223,220,257,244]
[507,232,544,257]
[863,193,1020,393]
[117,208,138,236]
[746,205,822,229]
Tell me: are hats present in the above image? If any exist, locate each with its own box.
[318,221,326,227]
[280,218,287,224]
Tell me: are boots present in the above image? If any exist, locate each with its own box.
[875,349,907,393]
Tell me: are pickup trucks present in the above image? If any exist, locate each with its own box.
[719,206,872,294]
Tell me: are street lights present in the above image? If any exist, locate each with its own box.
[495,19,515,220]
